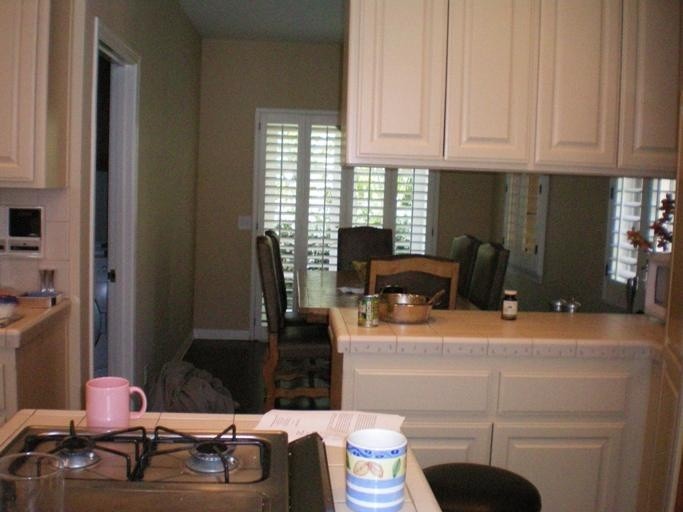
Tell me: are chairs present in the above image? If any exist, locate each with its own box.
[365,257,458,310]
[337,226,394,271]
[449,236,510,312]
[255,228,331,410]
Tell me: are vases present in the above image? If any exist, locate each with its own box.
[626,276,639,315]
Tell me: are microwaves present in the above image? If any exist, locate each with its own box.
[644,250,671,320]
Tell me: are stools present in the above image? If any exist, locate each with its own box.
[421,463,543,512]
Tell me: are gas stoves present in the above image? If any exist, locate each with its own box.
[0,423,338,512]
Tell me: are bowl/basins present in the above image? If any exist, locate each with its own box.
[377,292,441,322]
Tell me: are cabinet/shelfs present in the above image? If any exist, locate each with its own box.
[447,0,536,172]
[0,2,71,189]
[342,355,648,512]
[537,2,620,174]
[621,4,681,179]
[342,2,443,168]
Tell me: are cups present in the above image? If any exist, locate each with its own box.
[0,451,68,512]
[0,294,17,322]
[84,377,146,430]
[343,430,407,512]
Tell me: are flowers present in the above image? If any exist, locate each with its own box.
[626,191,677,273]
